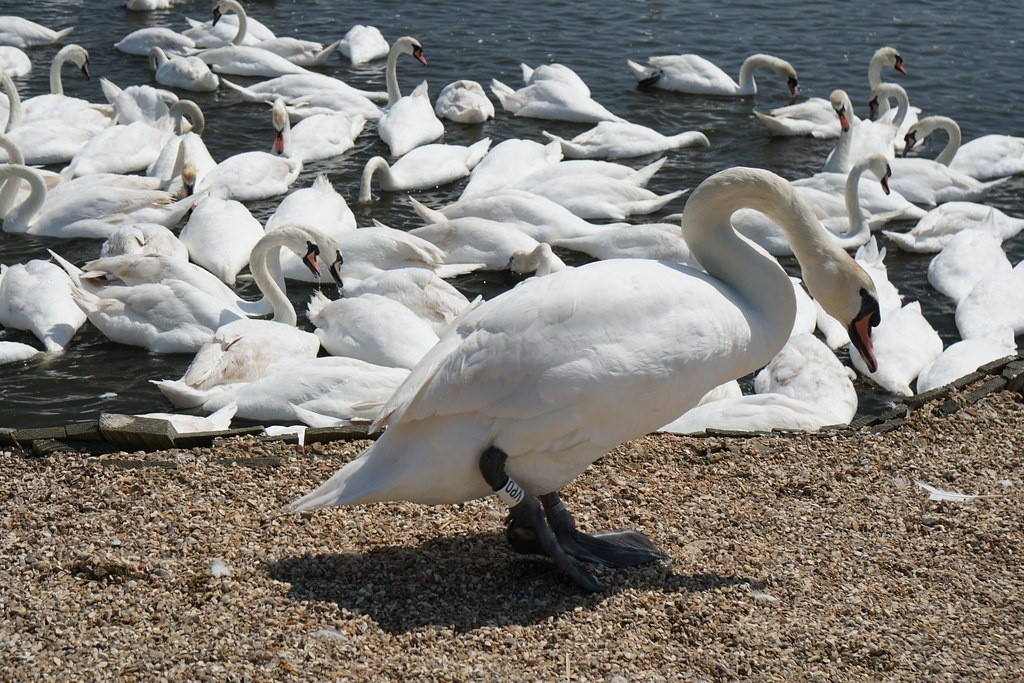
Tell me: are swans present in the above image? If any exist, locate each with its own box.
[0,0,1024,437]
[285,165,882,591]
